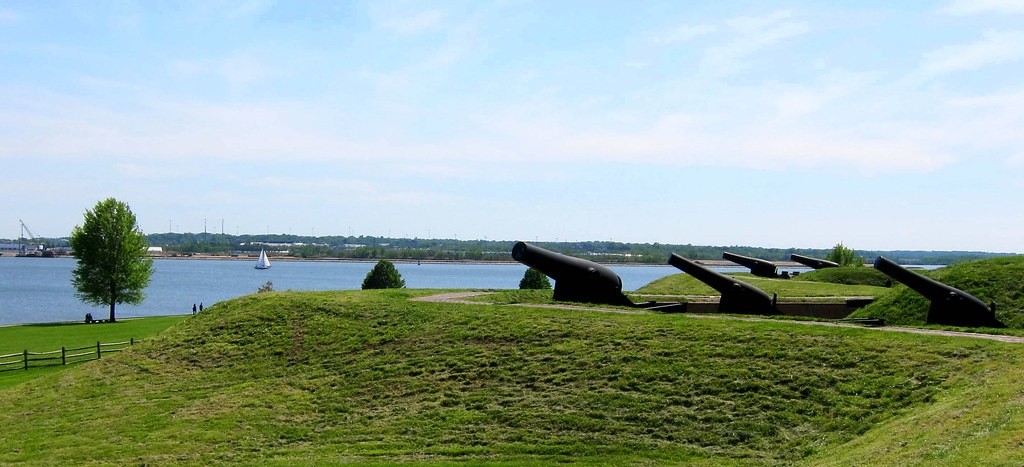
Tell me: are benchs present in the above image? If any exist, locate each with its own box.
[84,319,110,324]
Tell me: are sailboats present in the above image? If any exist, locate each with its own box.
[254,249,271,269]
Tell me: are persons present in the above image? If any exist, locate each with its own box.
[199,303,203,311]
[86,313,92,323]
[192,304,196,314]
